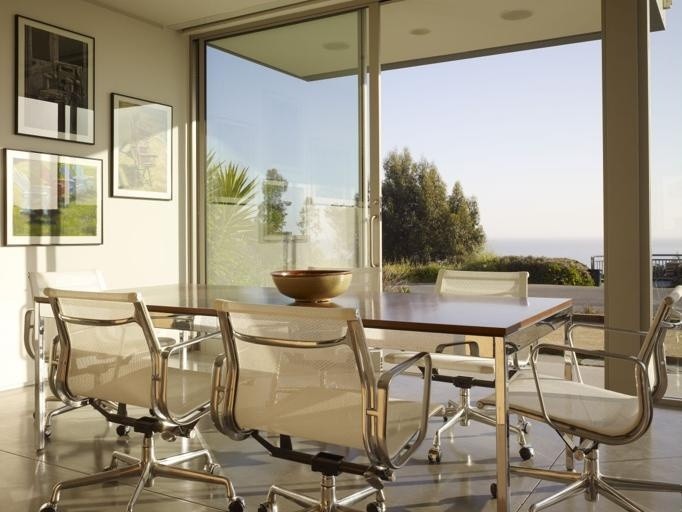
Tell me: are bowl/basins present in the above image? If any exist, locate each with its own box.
[270,269,353,302]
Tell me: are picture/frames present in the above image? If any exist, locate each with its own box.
[110,92,173,201]
[14,13,95,146]
[4,147,103,247]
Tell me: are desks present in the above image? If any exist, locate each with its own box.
[32,285,573,512]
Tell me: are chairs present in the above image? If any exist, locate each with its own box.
[384,268,539,464]
[38,288,246,512]
[23,272,132,436]
[475,283,682,511]
[211,298,432,511]
